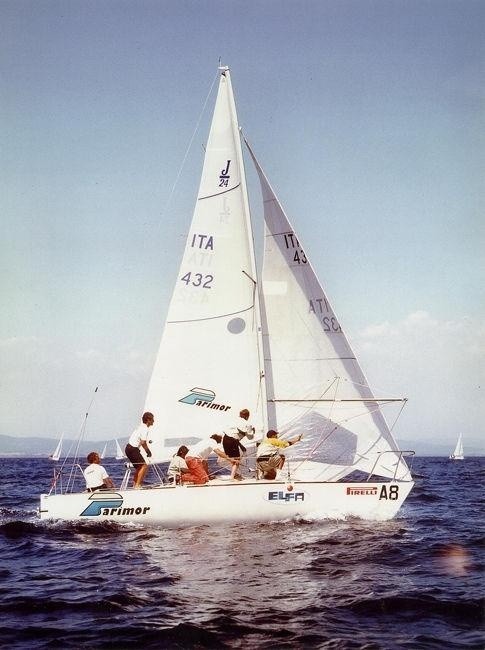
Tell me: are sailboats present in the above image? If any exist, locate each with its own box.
[36,53,417,528]
[447,431,469,461]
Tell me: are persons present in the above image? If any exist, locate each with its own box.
[176,433,240,484]
[222,408,255,482]
[167,445,191,484]
[82,451,117,493]
[256,430,304,482]
[124,411,155,489]
